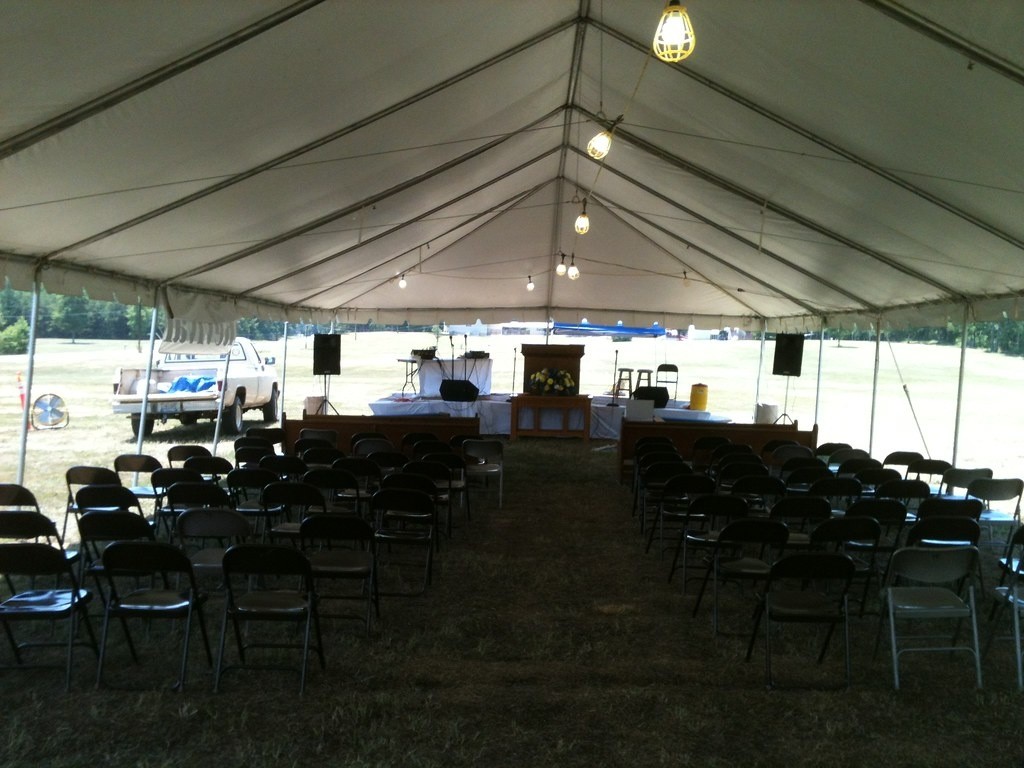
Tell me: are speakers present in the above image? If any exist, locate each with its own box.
[772,333,804,377]
[313,334,340,376]
[630,387,669,408]
[439,379,479,402]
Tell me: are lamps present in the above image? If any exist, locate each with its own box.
[584,115,624,160]
[556,254,567,276]
[526,276,535,291]
[399,274,407,289]
[652,0,697,63]
[574,198,590,234]
[568,254,581,280]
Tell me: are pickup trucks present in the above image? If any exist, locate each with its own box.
[110,336,280,437]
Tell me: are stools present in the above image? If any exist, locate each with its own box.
[616,367,634,399]
[636,369,652,389]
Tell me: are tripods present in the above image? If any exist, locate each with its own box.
[772,376,794,426]
[314,374,340,415]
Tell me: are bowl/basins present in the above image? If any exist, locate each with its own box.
[413,350,435,360]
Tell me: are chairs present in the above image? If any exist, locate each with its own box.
[629,435,1024,693]
[213,544,326,701]
[0,543,100,691]
[95,542,213,693]
[656,364,678,401]
[460,440,504,521]
[0,427,472,640]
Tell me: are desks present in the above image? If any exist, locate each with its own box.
[416,358,492,398]
[510,393,593,445]
[398,359,418,394]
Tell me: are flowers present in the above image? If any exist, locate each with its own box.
[530,368,575,395]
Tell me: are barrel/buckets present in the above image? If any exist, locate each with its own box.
[756,403,777,424]
[306,397,325,414]
[691,383,708,410]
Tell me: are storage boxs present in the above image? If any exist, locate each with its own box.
[624,400,655,423]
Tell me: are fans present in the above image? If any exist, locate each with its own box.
[31,394,70,431]
